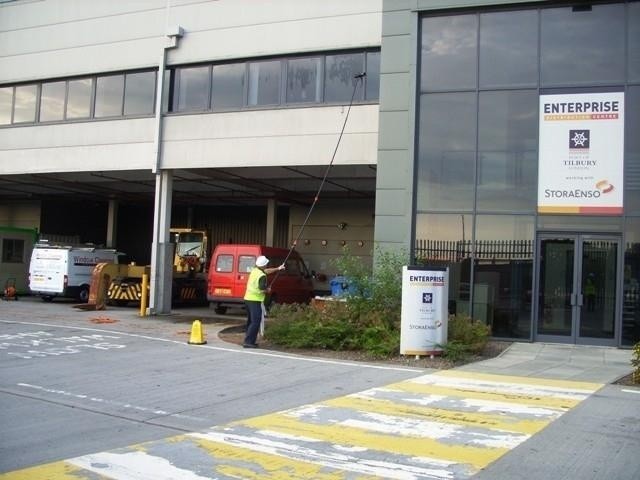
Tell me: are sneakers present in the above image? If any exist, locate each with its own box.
[243,342,257,348]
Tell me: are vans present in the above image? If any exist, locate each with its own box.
[206,244,317,315]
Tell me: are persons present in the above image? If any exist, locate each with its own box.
[241,255,287,349]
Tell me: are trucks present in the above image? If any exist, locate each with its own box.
[28,240,136,303]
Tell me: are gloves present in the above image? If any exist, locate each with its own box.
[279,263,285,270]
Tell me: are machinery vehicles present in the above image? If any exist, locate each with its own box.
[72,227,233,311]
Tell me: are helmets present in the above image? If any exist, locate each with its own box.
[256,256,269,266]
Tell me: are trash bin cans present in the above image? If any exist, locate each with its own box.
[330,275,375,298]
